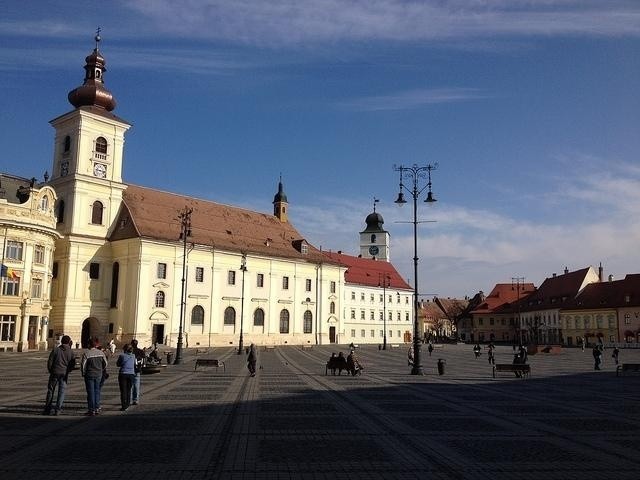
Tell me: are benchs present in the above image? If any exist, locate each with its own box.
[263,344,278,352]
[431,344,447,351]
[325,362,364,375]
[301,344,314,352]
[491,363,533,379]
[389,344,402,351]
[615,363,640,376]
[484,345,495,352]
[194,358,226,372]
[350,344,362,351]
[195,347,210,355]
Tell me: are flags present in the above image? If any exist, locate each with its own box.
[1,264,20,279]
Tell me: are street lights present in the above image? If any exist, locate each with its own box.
[391,161,440,374]
[512,276,525,352]
[173,206,194,365]
[237,248,250,355]
[377,271,394,348]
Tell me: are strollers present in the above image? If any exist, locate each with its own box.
[405,345,415,366]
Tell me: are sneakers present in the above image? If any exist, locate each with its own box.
[121,400,139,412]
[44,408,51,415]
[55,408,61,416]
[88,407,103,417]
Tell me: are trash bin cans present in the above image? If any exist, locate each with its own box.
[437,358,446,376]
[167,352,174,365]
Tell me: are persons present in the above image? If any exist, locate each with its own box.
[142,346,149,358]
[488,346,496,365]
[408,346,414,361]
[39,334,76,416]
[581,341,586,352]
[80,337,108,416]
[613,347,620,364]
[69,338,72,349]
[428,344,433,356]
[477,344,481,351]
[94,336,99,346]
[512,343,516,353]
[149,347,161,364]
[116,343,137,412]
[110,342,116,357]
[246,343,258,377]
[349,342,355,350]
[473,345,480,359]
[108,338,115,347]
[87,336,93,346]
[347,351,363,376]
[513,354,522,378]
[338,352,350,376]
[330,352,337,376]
[129,340,146,406]
[593,346,601,371]
[519,345,528,374]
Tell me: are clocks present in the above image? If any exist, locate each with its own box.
[60,162,69,176]
[94,163,107,178]
